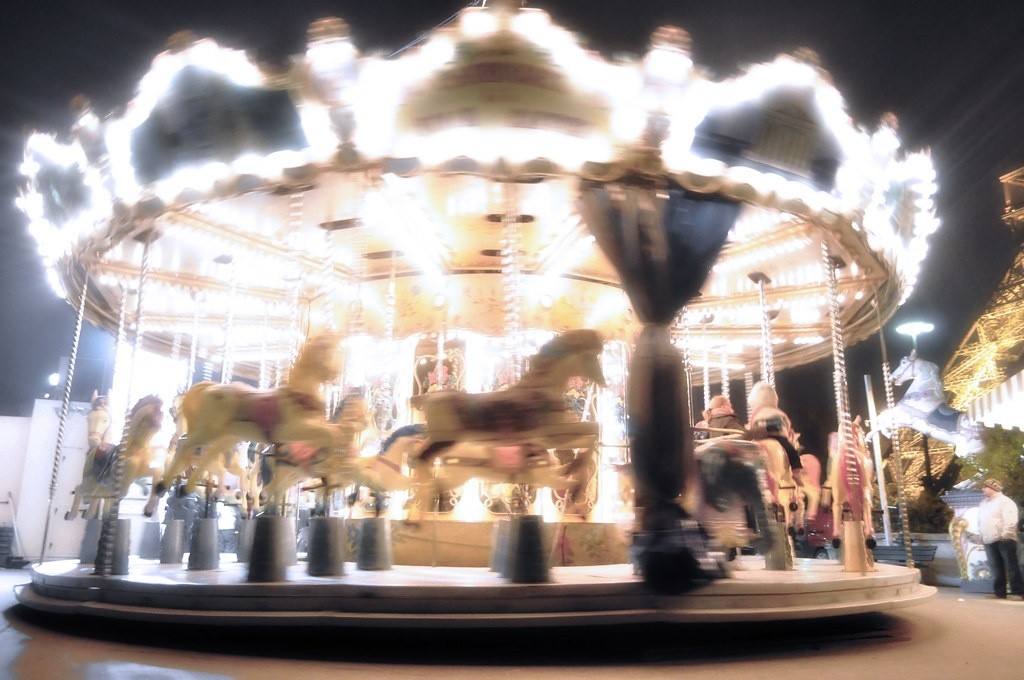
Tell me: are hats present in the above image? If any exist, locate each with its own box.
[984,478,1002,491]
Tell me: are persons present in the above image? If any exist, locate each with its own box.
[977,478,1024,600]
[695,382,805,489]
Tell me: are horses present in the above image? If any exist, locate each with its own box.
[63,328,881,550]
[864,349,999,493]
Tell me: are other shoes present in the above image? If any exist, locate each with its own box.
[986,593,1006,598]
[792,474,804,486]
